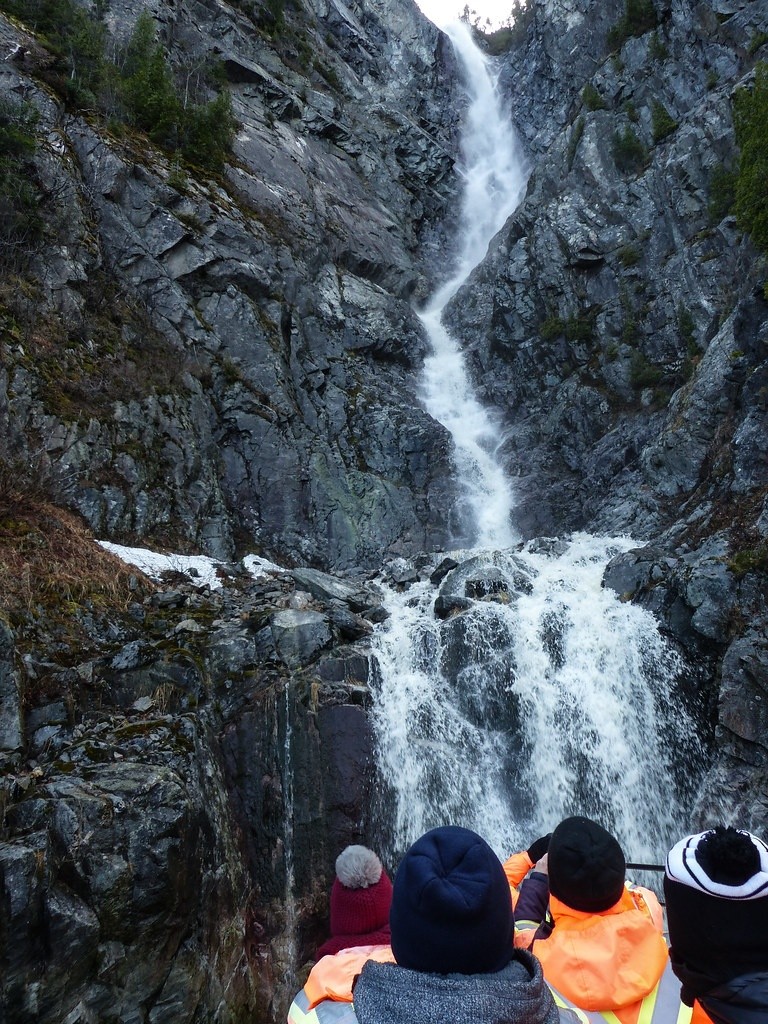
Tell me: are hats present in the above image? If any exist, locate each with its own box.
[662,823,768,1008]
[316,844,394,960]
[547,816,626,914]
[388,825,515,975]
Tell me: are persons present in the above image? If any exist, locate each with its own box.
[288,817,768,1024]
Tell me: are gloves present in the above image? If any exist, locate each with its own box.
[526,832,553,864]
